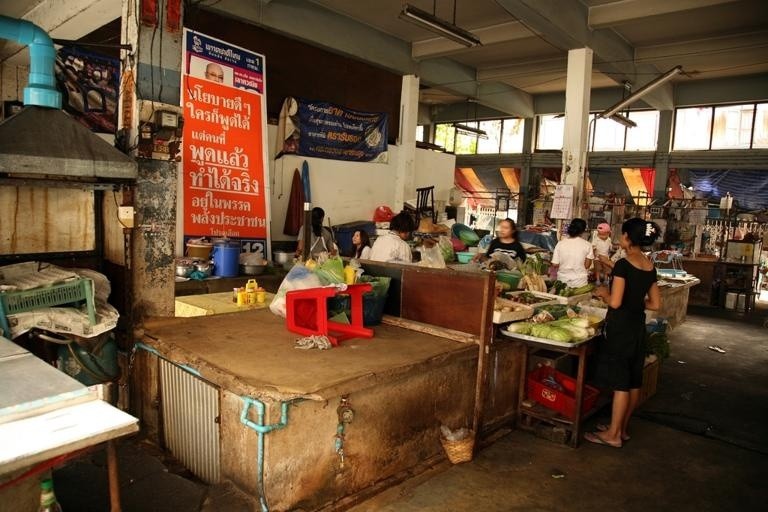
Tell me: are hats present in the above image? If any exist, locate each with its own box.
[597,223,611,233]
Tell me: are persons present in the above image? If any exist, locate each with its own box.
[295,207,338,262]
[583,217,662,447]
[204,62,226,86]
[597,245,627,280]
[473,218,527,265]
[369,213,414,266]
[350,229,372,261]
[592,222,613,286]
[551,216,597,288]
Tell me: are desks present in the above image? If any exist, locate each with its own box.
[1,334,30,359]
[0,354,140,511]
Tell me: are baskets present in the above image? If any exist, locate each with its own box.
[440,428,478,465]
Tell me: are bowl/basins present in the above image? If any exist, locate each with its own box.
[454,252,476,264]
[450,222,479,246]
[239,263,268,276]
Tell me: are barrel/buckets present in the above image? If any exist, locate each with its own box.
[185,243,212,256]
[209,246,240,277]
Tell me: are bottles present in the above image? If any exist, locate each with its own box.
[232,287,266,307]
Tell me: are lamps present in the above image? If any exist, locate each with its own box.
[599,64,683,119]
[451,121,486,135]
[396,5,479,50]
[608,113,637,127]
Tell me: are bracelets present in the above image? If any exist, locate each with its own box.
[608,261,612,266]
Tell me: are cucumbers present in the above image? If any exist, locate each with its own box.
[557,283,594,297]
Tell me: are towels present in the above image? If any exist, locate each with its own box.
[302,159,310,201]
[282,169,308,234]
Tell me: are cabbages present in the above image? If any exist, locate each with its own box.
[506,316,594,343]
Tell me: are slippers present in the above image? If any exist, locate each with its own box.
[596,423,631,441]
[585,431,621,448]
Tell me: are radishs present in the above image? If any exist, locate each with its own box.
[524,273,548,292]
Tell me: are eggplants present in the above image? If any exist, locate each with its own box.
[489,260,508,270]
